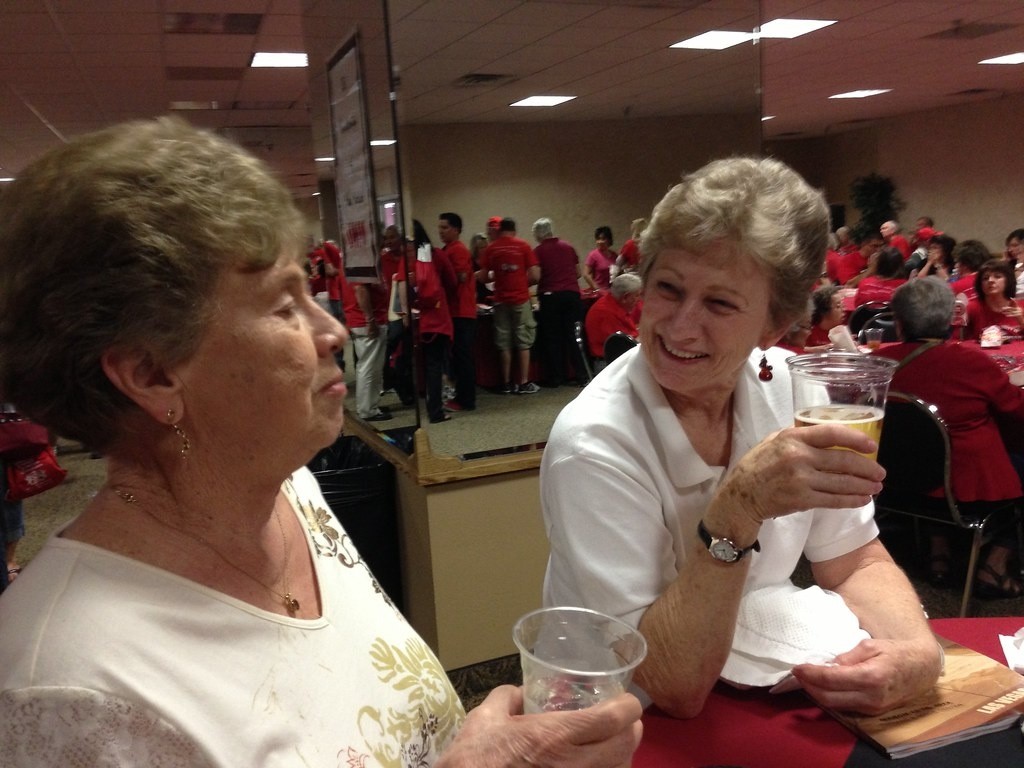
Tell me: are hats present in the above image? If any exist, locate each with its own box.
[487,216,503,230]
[719,584,873,687]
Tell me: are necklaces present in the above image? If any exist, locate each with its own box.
[105,483,301,612]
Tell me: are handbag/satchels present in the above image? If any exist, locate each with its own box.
[4,447,69,502]
[1,410,50,463]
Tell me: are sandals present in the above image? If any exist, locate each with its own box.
[972,564,1024,600]
[930,555,968,593]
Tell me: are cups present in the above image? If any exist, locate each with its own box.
[827,325,859,353]
[512,606,646,715]
[865,328,885,349]
[785,352,898,464]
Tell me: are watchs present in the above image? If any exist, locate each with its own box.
[697,518,760,562]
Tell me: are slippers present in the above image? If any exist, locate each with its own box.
[7,568,22,584]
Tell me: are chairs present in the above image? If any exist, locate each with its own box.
[849,301,896,344]
[572,320,639,384]
[874,391,1024,618]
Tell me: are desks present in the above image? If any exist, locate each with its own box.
[411,311,581,390]
[862,340,1024,370]
[632,617,1024,768]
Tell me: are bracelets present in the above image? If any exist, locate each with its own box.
[936,642,945,679]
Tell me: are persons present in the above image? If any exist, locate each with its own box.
[0,402,105,589]
[845,275,1024,597]
[0,115,642,768]
[526,159,944,720]
[773,216,1024,377]
[294,212,649,424]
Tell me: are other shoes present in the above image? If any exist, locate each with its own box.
[379,406,389,412]
[576,378,589,387]
[548,378,564,387]
[500,380,519,394]
[518,381,540,394]
[442,399,466,412]
[362,413,392,421]
[430,413,451,423]
